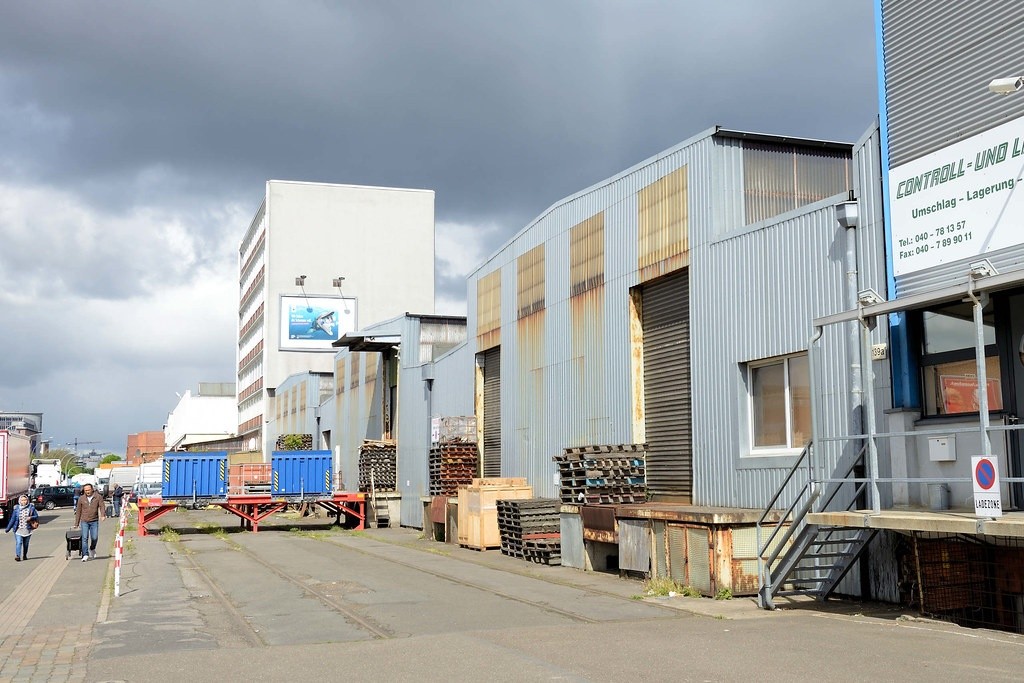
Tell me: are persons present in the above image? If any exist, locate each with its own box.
[74,484,81,515]
[5,495,38,561]
[74,484,106,562]
[111,483,122,517]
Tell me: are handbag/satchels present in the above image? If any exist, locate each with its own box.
[30,508,39,529]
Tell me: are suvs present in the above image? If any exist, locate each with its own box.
[33,485,81,512]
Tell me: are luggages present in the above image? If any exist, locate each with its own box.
[66,525,83,557]
[107,505,113,518]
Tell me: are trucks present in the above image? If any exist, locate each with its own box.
[30,459,63,486]
[0,429,38,524]
[68,463,162,507]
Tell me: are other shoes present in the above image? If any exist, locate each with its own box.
[23,556,27,560]
[89,547,97,558]
[81,555,89,562]
[112,512,119,517]
[15,555,20,561]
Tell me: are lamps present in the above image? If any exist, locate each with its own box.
[857,288,886,306]
[336,276,345,287]
[969,258,999,279]
[300,275,307,285]
